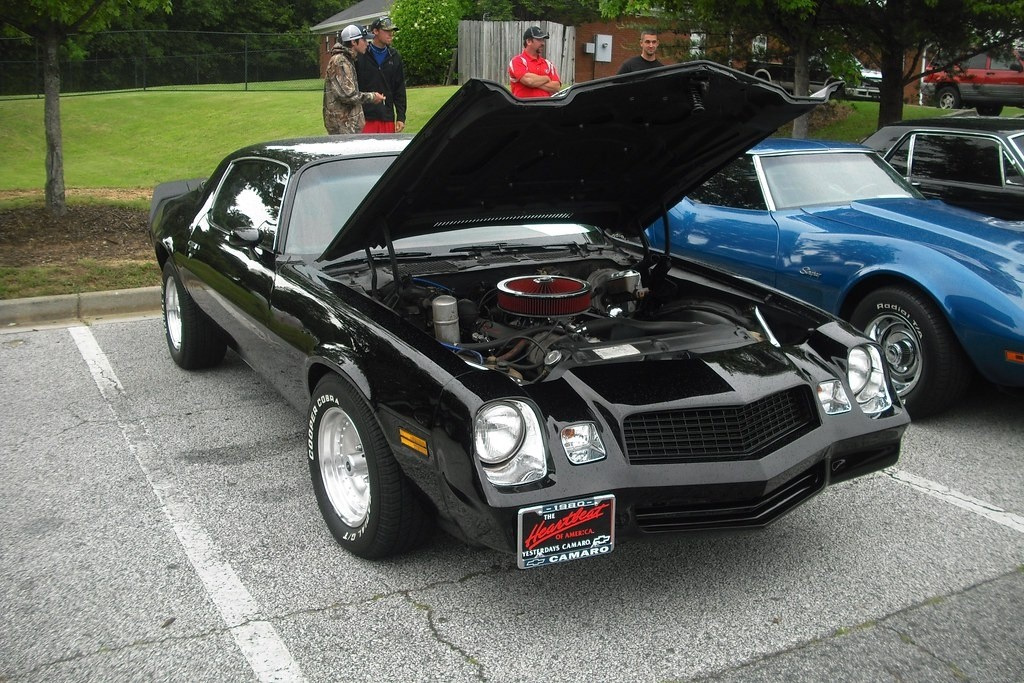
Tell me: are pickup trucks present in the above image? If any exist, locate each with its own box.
[746,49,883,103]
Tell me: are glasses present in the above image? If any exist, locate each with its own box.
[375,16,391,28]
[350,29,368,38]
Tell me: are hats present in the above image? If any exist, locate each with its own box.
[524,26,549,40]
[341,24,375,42]
[371,18,399,31]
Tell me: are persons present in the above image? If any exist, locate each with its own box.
[323,26,386,134]
[507,26,561,99]
[354,17,407,134]
[616,29,665,75]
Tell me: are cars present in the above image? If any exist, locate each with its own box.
[858,117,1024,223]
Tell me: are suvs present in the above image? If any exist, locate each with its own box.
[921,46,1024,116]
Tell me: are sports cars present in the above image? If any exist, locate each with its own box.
[641,137,1024,421]
[144,60,911,572]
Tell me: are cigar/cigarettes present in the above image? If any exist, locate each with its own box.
[382,93,385,105]
